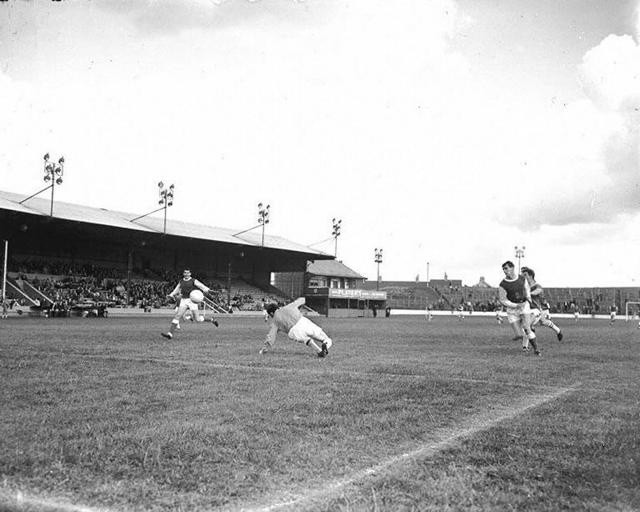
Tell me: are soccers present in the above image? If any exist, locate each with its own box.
[190,290,203,303]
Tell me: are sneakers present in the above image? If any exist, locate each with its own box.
[317,351,326,358]
[557,329,563,341]
[161,332,173,340]
[523,346,529,352]
[534,351,542,356]
[322,343,328,355]
[213,319,218,327]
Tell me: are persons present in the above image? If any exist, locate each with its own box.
[372,260,640,357]
[260,296,333,357]
[0,259,285,340]
